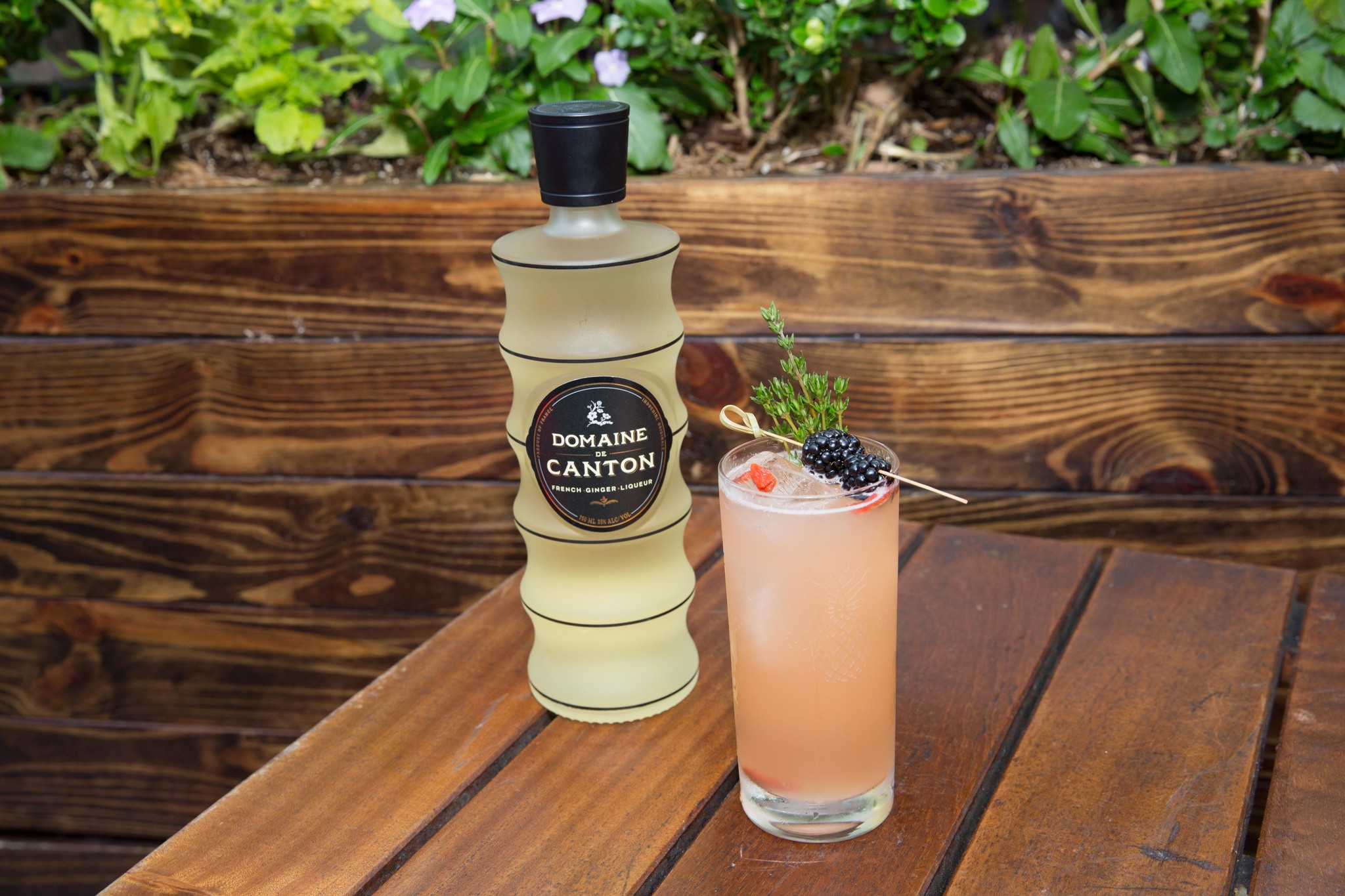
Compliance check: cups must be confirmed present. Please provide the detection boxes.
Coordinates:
[717,431,900,847]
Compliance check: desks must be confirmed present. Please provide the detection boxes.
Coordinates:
[97,490,1345,896]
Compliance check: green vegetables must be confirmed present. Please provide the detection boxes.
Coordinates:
[748,301,850,469]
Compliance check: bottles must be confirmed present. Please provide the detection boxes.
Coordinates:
[489,99,701,726]
